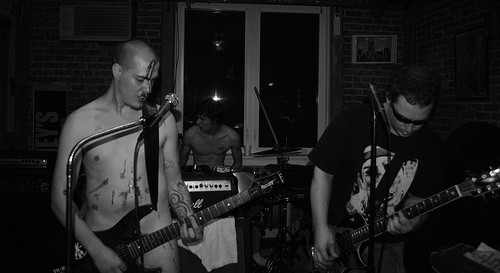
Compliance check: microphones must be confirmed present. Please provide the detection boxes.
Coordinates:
[369,82,390,128]
[138,93,179,141]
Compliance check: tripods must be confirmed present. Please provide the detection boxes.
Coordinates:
[264,159,298,273]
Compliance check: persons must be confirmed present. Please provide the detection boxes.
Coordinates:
[308,63,447,273]
[179,97,273,270]
[51,39,204,273]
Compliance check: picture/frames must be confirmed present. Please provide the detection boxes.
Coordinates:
[352,34,398,64]
[452,26,489,103]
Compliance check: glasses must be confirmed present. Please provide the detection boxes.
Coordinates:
[391,103,429,125]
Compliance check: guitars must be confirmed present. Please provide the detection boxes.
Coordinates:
[273,164,500,273]
[42,163,290,273]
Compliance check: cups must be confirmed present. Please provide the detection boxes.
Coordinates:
[243,145,251,156]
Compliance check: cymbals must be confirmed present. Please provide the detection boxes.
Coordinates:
[252,147,302,155]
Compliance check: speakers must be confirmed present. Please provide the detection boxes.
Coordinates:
[0,192,68,273]
